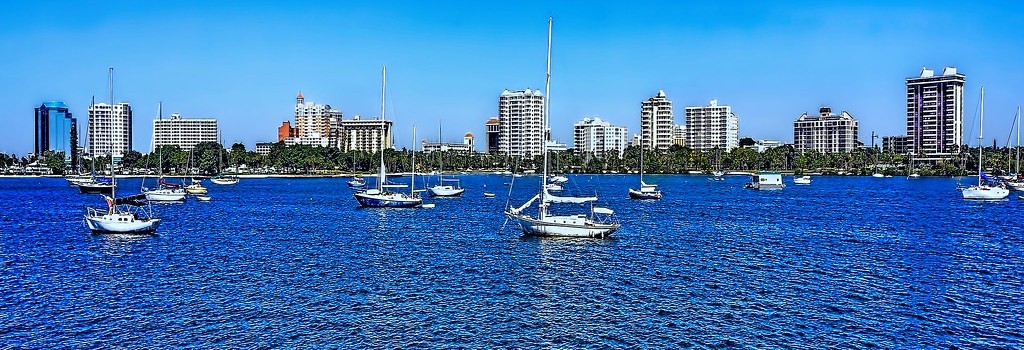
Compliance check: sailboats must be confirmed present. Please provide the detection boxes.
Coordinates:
[346,66,464,208]
[907,148,920,180]
[65,95,118,194]
[956,86,1024,200]
[629,148,662,199]
[494,18,620,240]
[140,101,211,201]
[83,67,162,235]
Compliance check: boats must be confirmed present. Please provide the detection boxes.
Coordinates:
[484,192,495,196]
[794,176,811,184]
[872,167,892,178]
[210,178,240,185]
